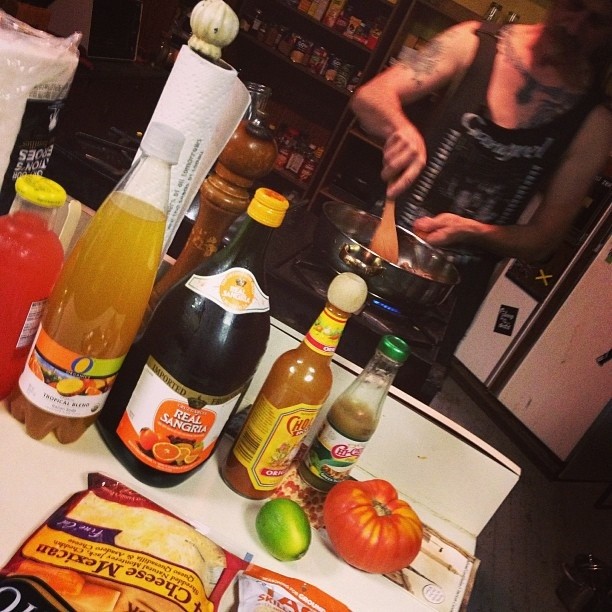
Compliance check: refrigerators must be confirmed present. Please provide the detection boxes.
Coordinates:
[439,166,612,481]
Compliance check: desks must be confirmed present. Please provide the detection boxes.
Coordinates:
[1,180,521,612]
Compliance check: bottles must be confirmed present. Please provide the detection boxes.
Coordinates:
[248,7,266,34]
[319,53,335,77]
[272,22,288,48]
[333,5,354,34]
[290,37,309,64]
[296,335,408,492]
[291,144,316,174]
[301,41,316,67]
[11,124,184,446]
[278,31,297,55]
[220,273,368,499]
[297,0,312,13]
[366,25,381,49]
[352,21,368,43]
[322,0,346,29]
[346,63,366,94]
[260,124,276,140]
[272,126,300,168]
[308,46,324,68]
[334,64,351,87]
[324,56,342,81]
[312,52,328,71]
[0,175,68,405]
[298,146,324,183]
[313,0,330,22]
[307,0,320,16]
[344,16,361,38]
[97,188,289,487]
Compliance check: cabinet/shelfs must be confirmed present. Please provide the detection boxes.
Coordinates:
[133,0,404,202]
[306,0,487,230]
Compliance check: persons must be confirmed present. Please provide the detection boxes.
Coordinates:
[350,2,612,335]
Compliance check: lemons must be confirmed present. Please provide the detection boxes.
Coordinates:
[256,498,311,562]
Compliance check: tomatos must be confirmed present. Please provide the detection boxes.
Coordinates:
[323,479,423,575]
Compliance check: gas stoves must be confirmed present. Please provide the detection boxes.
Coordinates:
[295,245,449,347]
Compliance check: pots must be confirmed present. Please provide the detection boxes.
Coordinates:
[321,202,498,319]
